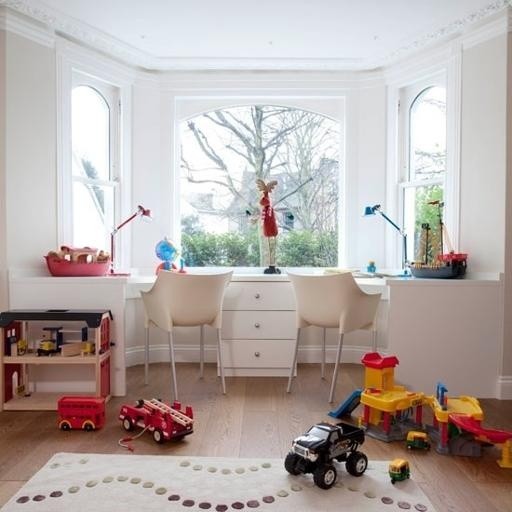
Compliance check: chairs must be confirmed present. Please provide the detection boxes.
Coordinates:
[139,270,234,404]
[285,271,383,404]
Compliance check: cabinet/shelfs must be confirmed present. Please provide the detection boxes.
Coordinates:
[0,309,113,412]
[218,274,298,377]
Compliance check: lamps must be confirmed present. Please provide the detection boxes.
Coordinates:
[108,204,153,276]
[364,204,412,277]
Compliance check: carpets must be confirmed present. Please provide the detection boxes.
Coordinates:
[0,452,439,512]
